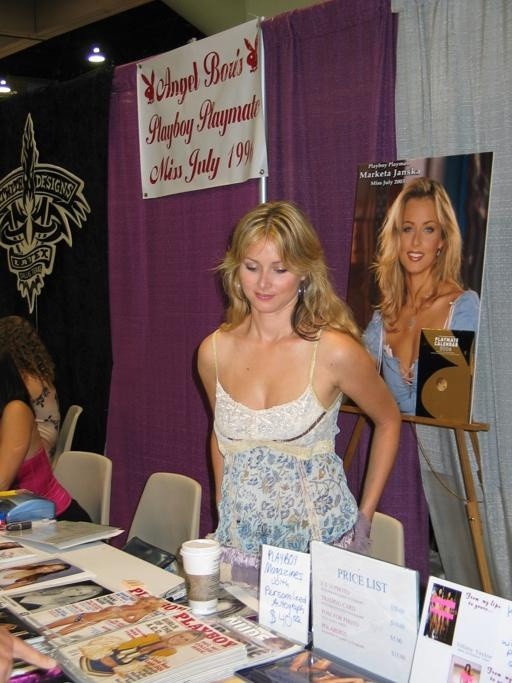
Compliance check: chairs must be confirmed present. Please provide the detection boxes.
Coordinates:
[123,472,203,561]
[52,450,113,544]
[51,404,83,471]
[366,511,406,567]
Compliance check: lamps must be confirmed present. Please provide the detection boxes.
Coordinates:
[87,45,107,62]
[0,79,11,93]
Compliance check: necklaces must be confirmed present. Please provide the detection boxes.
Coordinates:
[406,304,427,329]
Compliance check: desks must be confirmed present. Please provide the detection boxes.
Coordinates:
[0,531,185,602]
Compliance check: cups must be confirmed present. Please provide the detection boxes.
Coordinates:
[178,537,221,617]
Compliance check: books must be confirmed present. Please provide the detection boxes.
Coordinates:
[1,520,393,682]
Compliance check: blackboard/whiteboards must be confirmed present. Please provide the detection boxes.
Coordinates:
[257,540,511,682]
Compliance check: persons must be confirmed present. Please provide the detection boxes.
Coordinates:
[362,174,480,417]
[0,314,62,462]
[0,349,94,523]
[457,662,474,682]
[195,200,403,586]
[439,591,452,634]
[432,587,444,636]
